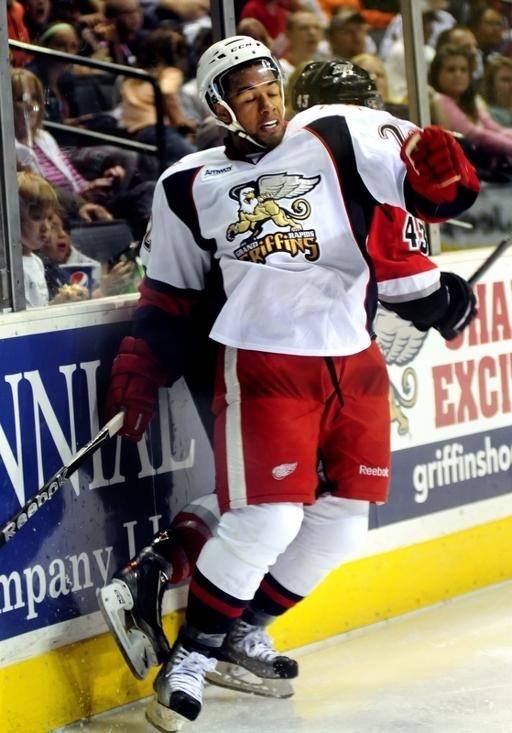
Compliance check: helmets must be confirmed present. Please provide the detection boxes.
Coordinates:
[292,57,384,112]
[196,35,287,102]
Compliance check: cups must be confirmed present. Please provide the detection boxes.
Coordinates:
[59,263,93,301]
[122,248,146,293]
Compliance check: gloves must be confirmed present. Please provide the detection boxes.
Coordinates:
[107,335,161,442]
[432,269,476,345]
[399,126,481,218]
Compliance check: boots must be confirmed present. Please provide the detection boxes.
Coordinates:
[111,526,175,664]
[152,627,219,722]
[222,607,299,679]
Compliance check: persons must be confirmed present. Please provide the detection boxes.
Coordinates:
[10,1,511,311]
